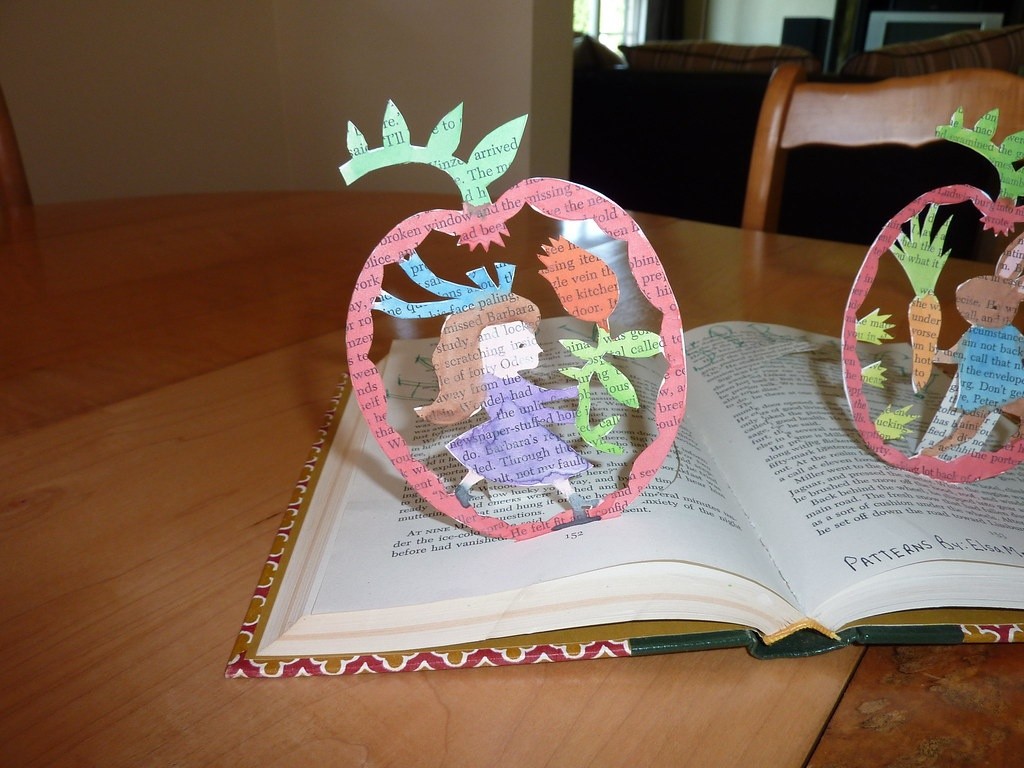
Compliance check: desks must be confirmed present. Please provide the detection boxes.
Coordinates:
[0,192,1022,768]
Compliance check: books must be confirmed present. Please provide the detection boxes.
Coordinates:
[220,306,1024,679]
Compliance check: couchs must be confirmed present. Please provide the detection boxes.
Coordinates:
[571,64,1023,260]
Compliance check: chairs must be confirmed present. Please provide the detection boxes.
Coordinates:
[742,60,1024,237]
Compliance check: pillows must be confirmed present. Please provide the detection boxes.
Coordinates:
[571,30,622,65]
[838,24,1023,81]
[619,38,823,80]
